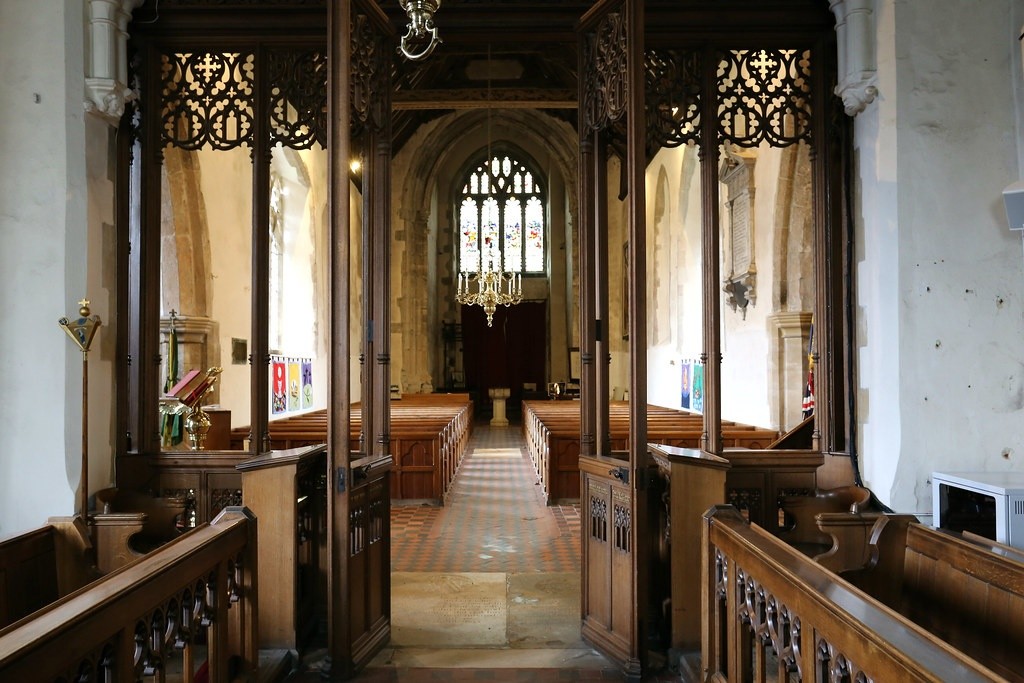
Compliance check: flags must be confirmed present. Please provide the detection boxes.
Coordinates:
[801,313,815,422]
[160,329,184,446]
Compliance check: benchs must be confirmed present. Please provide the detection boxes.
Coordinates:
[522,400,779,505]
[229,393,471,505]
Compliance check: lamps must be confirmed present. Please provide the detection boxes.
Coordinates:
[454,45,540,391]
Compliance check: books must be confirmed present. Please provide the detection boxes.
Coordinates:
[165,370,216,407]
